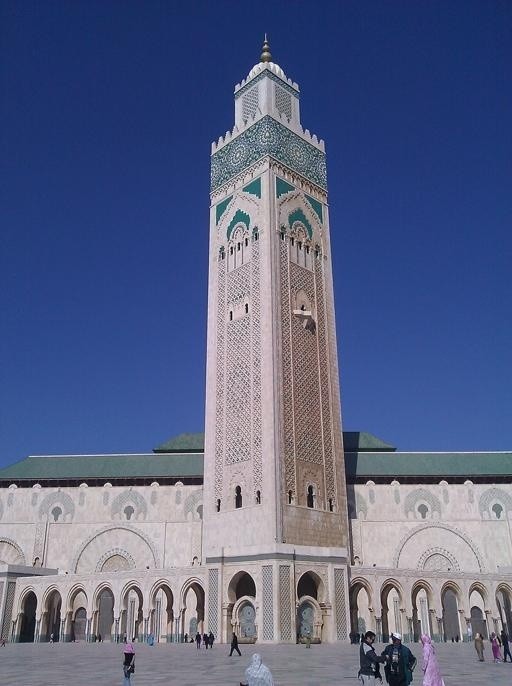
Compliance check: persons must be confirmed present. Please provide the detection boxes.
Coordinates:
[350,631,445,686]
[305,630,312,648]
[147,632,155,646]
[97,633,102,642]
[245,653,275,686]
[474,630,511,662]
[229,632,242,656]
[1,637,6,648]
[119,644,135,686]
[48,633,56,647]
[124,632,128,643]
[185,632,215,649]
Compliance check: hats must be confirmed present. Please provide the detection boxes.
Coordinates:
[390,632,402,640]
